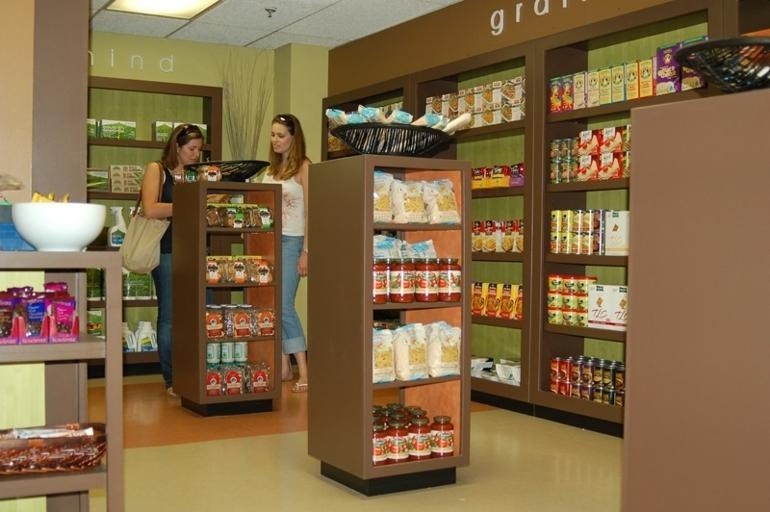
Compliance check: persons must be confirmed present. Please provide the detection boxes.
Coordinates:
[262,113,313,393]
[141,123,204,398]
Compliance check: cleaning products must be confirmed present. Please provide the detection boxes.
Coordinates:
[107,206,129,247]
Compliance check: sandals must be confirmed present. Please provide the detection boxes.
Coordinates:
[292,382,308,393]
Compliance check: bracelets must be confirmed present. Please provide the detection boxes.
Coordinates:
[300,248,309,254]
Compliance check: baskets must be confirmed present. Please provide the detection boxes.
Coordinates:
[330,122,450,157]
[674,37,770,94]
[184,160,270,181]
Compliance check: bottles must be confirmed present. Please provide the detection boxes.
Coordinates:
[206,303,275,340]
[206,362,274,397]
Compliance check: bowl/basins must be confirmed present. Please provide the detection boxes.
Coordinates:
[11,202,107,253]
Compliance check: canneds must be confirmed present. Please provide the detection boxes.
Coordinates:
[546,274,597,330]
[206,254,273,284]
[372,404,454,462]
[548,207,606,255]
[372,259,463,305]
[551,139,579,186]
[206,201,273,229]
[206,304,274,338]
[548,352,630,409]
[206,342,248,365]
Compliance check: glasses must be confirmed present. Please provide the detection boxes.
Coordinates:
[180,125,199,138]
[277,114,293,127]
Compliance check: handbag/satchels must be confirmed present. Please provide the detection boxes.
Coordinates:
[119,211,172,275]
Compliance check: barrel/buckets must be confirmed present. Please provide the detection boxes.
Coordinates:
[122,318,158,353]
